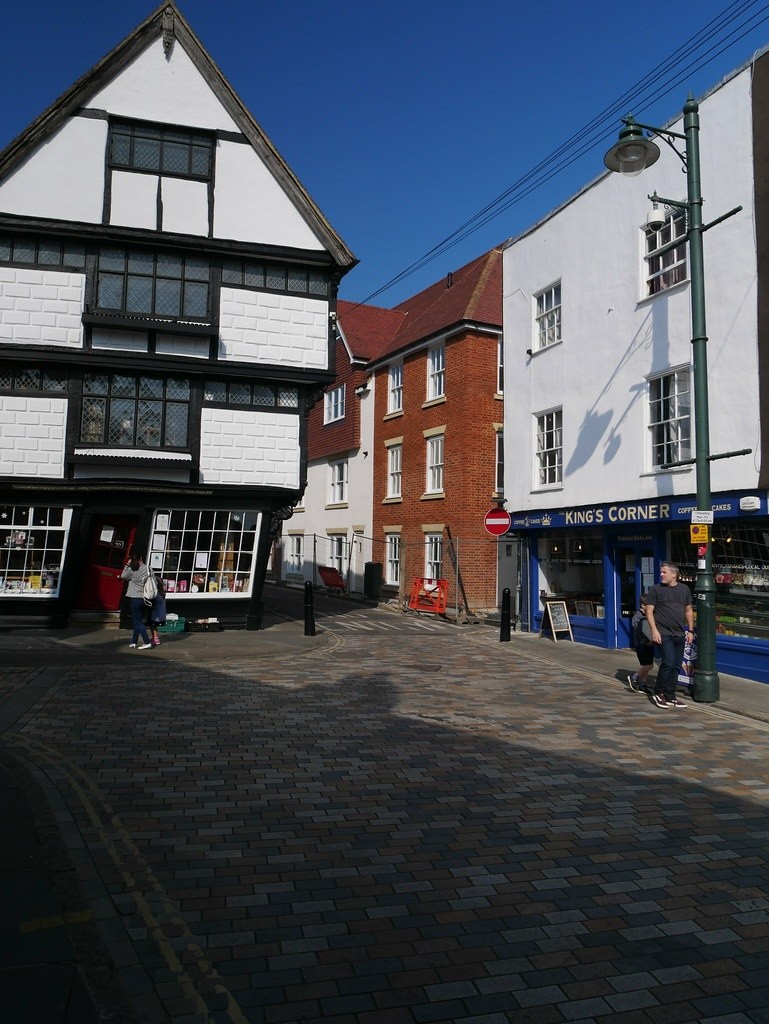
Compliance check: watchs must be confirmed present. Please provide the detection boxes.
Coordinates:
[688,630,695,635]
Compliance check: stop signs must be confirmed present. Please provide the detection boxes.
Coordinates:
[483,507,512,536]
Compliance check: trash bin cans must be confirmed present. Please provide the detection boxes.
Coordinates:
[364,562,382,598]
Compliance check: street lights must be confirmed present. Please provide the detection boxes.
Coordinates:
[601,87,726,704]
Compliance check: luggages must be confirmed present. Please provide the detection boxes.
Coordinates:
[152,637,160,644]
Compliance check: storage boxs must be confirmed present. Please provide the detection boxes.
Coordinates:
[218,560,234,580]
[219,542,233,560]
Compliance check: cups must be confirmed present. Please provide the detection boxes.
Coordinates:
[739,617,745,622]
[745,618,750,623]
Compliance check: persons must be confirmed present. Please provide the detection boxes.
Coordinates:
[121,550,154,650]
[645,561,694,709]
[145,576,166,645]
[626,592,656,695]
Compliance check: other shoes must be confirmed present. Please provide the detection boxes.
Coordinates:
[638,684,654,694]
[627,675,637,693]
[666,698,687,707]
[129,643,137,648]
[652,694,669,709]
[138,643,152,650]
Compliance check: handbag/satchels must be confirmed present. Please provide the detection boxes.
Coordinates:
[143,566,158,606]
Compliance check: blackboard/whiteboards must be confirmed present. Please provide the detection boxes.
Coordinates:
[541,601,573,631]
